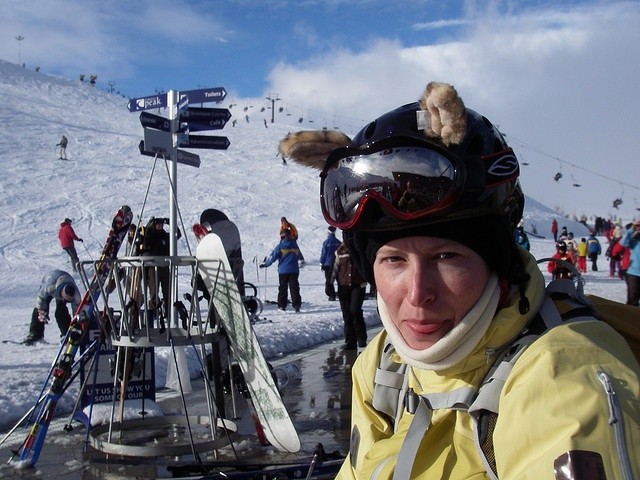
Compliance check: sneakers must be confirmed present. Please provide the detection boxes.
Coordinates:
[343,344,356,349]
[329,296,334,300]
[61,336,67,344]
[359,340,365,347]
[26,332,44,342]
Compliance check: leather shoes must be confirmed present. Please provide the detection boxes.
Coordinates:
[105,278,116,293]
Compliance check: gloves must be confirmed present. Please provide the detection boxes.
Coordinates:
[298,263,302,267]
[38,309,50,324]
[259,262,266,268]
[320,262,323,272]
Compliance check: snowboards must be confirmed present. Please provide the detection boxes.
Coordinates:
[200,209,230,419]
[211,220,257,436]
[193,232,302,453]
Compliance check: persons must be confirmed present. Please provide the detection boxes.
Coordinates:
[56,135,68,159]
[59,218,84,273]
[25,268,82,345]
[280,217,298,241]
[320,225,342,302]
[259,228,305,314]
[142,215,182,319]
[547,218,640,305]
[278,82,639,479]
[328,240,368,350]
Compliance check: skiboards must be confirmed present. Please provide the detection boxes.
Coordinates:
[17,204,133,468]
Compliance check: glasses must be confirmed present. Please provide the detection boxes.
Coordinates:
[559,243,564,246]
[319,134,520,229]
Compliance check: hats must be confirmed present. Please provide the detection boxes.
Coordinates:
[556,241,567,253]
[328,226,335,233]
[128,224,136,228]
[280,229,290,238]
[63,282,75,302]
[277,81,531,315]
